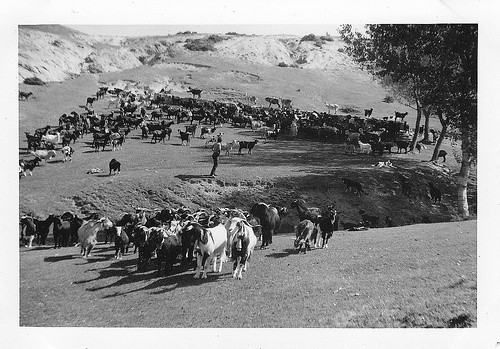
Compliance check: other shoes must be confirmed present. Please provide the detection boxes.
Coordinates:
[211,174,215,177]
[114,171,117,174]
[118,171,120,173]
[214,173,218,175]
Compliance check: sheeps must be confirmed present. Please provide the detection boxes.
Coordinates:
[428,181,442,204]
[400,179,411,196]
[342,178,364,197]
[19,198,399,281]
[19,85,448,178]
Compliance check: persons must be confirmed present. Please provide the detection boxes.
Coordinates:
[209,137,222,178]
